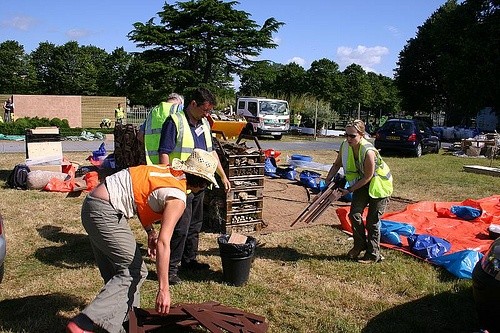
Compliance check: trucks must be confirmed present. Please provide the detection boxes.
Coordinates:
[211,96,294,140]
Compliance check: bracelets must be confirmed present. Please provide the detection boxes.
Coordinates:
[347,187,353,193]
[146,228,154,234]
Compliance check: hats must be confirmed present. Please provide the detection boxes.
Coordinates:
[171,148,219,189]
[168,93,182,104]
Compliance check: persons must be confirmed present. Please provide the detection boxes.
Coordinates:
[158,87,231,284]
[294,112,301,127]
[3,98,14,122]
[136,93,184,165]
[67,148,220,333]
[325,119,393,264]
[115,103,124,123]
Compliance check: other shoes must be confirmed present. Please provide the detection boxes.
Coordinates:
[347,244,365,259]
[169,273,181,285]
[358,256,375,264]
[66,315,96,333]
[182,258,210,271]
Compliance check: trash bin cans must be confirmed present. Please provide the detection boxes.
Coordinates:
[218,234,255,286]
[207,114,247,139]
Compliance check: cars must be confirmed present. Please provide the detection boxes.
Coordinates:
[373,118,442,158]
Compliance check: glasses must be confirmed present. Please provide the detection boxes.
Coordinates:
[345,133,360,138]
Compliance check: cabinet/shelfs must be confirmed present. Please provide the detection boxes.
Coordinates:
[208,146,268,233]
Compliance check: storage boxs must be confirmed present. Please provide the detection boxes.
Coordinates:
[28,164,62,172]
